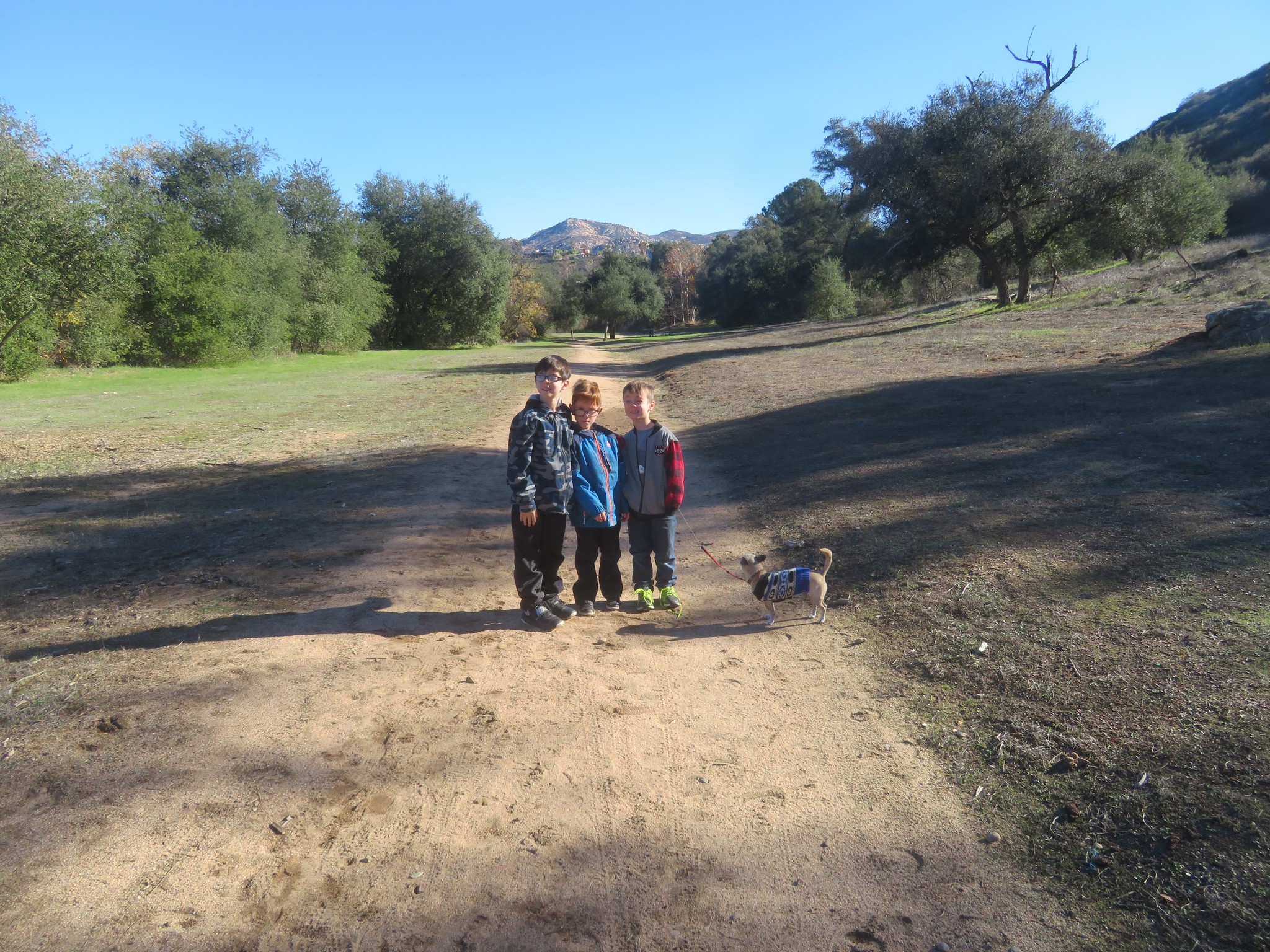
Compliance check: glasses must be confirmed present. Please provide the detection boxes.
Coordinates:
[535,375,565,382]
[572,404,600,417]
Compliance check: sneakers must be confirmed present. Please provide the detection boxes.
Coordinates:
[631,586,655,611]
[605,597,622,610]
[521,600,564,632]
[576,599,595,615]
[545,594,578,620]
[659,586,680,609]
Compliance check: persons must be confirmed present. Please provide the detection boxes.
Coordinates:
[507,356,576,631]
[568,378,626,616]
[622,381,685,611]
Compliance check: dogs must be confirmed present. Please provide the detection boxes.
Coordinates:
[740,548,833,626]
